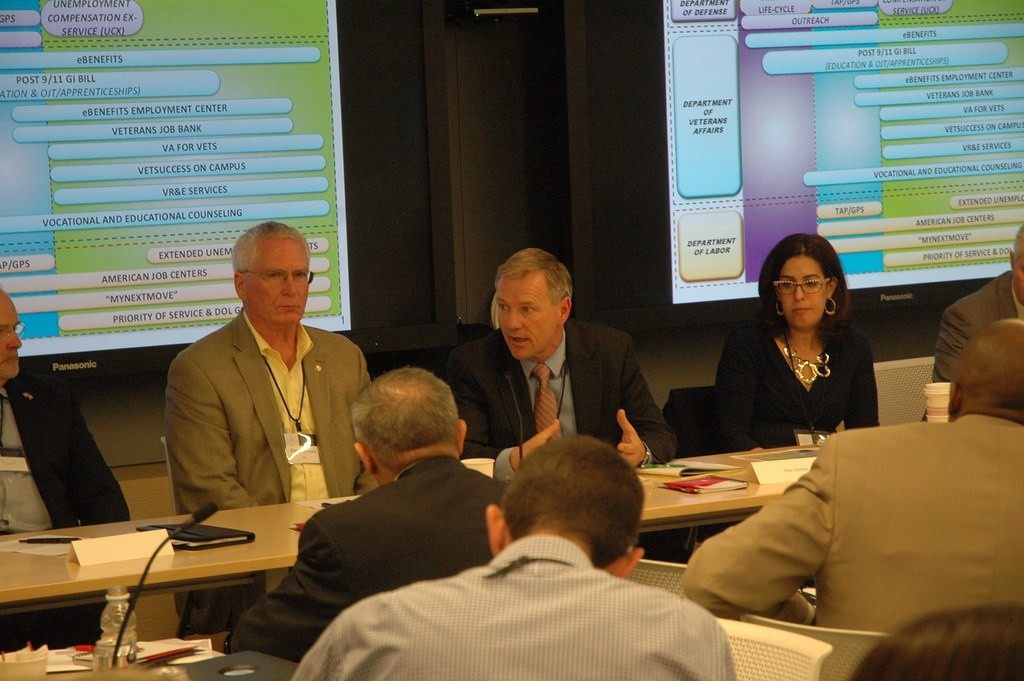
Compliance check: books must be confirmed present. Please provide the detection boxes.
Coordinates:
[139,523,256,551]
[666,476,748,494]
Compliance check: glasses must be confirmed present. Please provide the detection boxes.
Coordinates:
[773,279,828,294]
[0,324,24,337]
[241,269,313,287]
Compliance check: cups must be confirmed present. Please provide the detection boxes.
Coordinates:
[0,650,47,681]
[460,458,495,480]
[923,383,950,422]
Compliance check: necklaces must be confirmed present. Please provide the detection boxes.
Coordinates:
[784,345,831,384]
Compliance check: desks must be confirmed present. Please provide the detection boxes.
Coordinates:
[0,446,823,625]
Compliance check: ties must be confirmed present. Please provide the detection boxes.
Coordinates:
[532,364,557,433]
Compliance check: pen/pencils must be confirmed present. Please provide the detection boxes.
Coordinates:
[76,644,140,652]
[640,463,687,468]
[19,538,81,544]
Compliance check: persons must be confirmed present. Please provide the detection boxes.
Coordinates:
[227,369,508,663]
[294,434,738,681]
[446,247,680,467]
[713,233,879,453]
[685,319,1024,635]
[165,222,380,635]
[0,287,130,654]
[932,224,1024,384]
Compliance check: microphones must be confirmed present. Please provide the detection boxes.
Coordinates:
[112,501,220,666]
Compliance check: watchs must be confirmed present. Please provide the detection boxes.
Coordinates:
[641,452,649,468]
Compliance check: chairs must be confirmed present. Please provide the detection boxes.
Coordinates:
[876,349,943,425]
[623,555,890,680]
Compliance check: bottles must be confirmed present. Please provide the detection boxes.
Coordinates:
[101,584,137,642]
